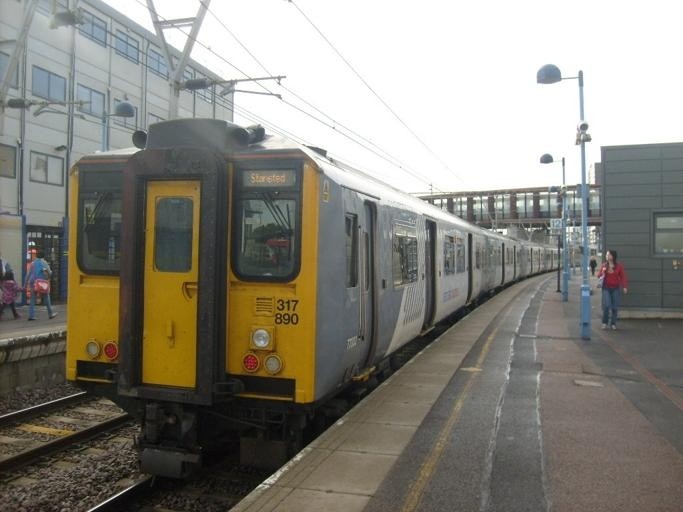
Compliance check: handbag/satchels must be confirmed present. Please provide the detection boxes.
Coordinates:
[33,277,51,295]
[596,274,605,288]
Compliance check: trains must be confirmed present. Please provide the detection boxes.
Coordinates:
[66,119,564,488]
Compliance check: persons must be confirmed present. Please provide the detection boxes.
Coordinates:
[589,255,597,277]
[597,248,629,330]
[0,268,26,319]
[23,250,58,321]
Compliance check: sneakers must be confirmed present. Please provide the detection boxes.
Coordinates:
[0,312,59,321]
[601,323,617,330]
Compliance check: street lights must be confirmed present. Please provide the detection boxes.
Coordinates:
[537,63,591,342]
[101,93,134,152]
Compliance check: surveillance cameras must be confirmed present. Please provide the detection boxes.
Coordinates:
[578,121,589,132]
